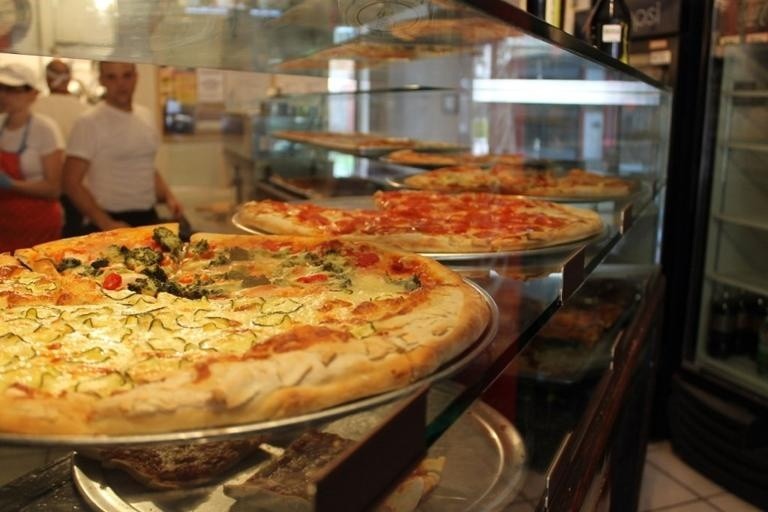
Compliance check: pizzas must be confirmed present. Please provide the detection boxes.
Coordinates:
[0,222,492,435]
[388,147,526,165]
[374,456,445,512]
[539,303,623,347]
[237,191,603,253]
[76,426,359,512]
[404,163,631,198]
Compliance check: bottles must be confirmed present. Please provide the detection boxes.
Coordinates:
[590,1,632,66]
[707,289,767,378]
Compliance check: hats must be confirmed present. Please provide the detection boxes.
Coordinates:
[0,64,42,93]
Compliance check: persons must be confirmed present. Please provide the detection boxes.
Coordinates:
[61,61,184,236]
[34,58,95,150]
[0,63,65,254]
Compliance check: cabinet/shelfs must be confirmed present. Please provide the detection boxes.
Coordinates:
[0,0,768,511]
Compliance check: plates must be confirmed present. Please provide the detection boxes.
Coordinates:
[0,275,502,447]
[231,193,612,261]
[71,380,531,511]
[386,170,650,203]
[504,278,643,386]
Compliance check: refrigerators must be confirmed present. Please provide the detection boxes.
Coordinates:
[667,0,768,512]
